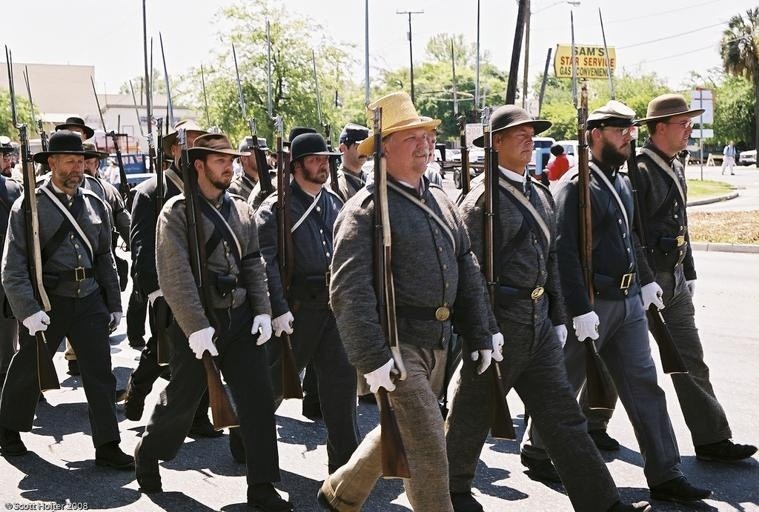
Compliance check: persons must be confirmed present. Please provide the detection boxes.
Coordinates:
[0,90,758,511]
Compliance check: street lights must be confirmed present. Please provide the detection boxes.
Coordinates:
[520,0,584,111]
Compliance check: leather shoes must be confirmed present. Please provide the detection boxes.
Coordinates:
[1,396,758,510]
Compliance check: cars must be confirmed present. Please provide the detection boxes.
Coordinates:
[686,141,758,167]
[9,123,589,196]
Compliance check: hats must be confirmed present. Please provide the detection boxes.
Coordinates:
[0,91,705,160]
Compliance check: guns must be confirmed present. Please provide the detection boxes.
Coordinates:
[1,1,690,479]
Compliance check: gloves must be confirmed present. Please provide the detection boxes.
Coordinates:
[21,279,696,393]
